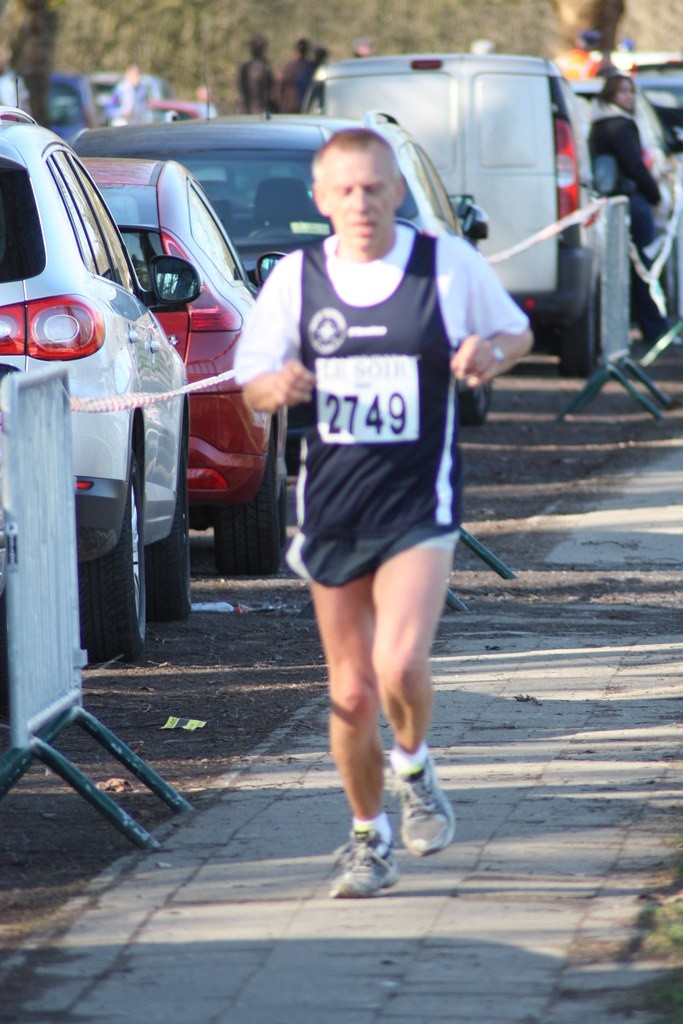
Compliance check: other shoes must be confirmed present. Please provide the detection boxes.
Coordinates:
[643,333,683,347]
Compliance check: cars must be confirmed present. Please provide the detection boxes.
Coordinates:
[70,159,296,586]
[45,75,210,131]
[552,48,683,153]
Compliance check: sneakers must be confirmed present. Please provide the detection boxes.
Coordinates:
[388,752,455,857]
[328,826,400,899]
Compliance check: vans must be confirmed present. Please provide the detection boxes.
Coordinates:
[298,52,611,381]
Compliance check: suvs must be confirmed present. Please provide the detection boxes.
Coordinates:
[56,106,499,424]
[1,107,201,657]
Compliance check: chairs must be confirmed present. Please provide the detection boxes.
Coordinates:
[252,176,314,227]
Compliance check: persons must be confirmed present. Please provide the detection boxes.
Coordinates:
[353,35,374,57]
[193,87,218,118]
[232,128,533,899]
[587,74,683,346]
[277,38,328,114]
[554,30,602,78]
[104,64,151,128]
[237,37,274,114]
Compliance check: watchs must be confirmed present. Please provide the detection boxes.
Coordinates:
[491,344,504,363]
